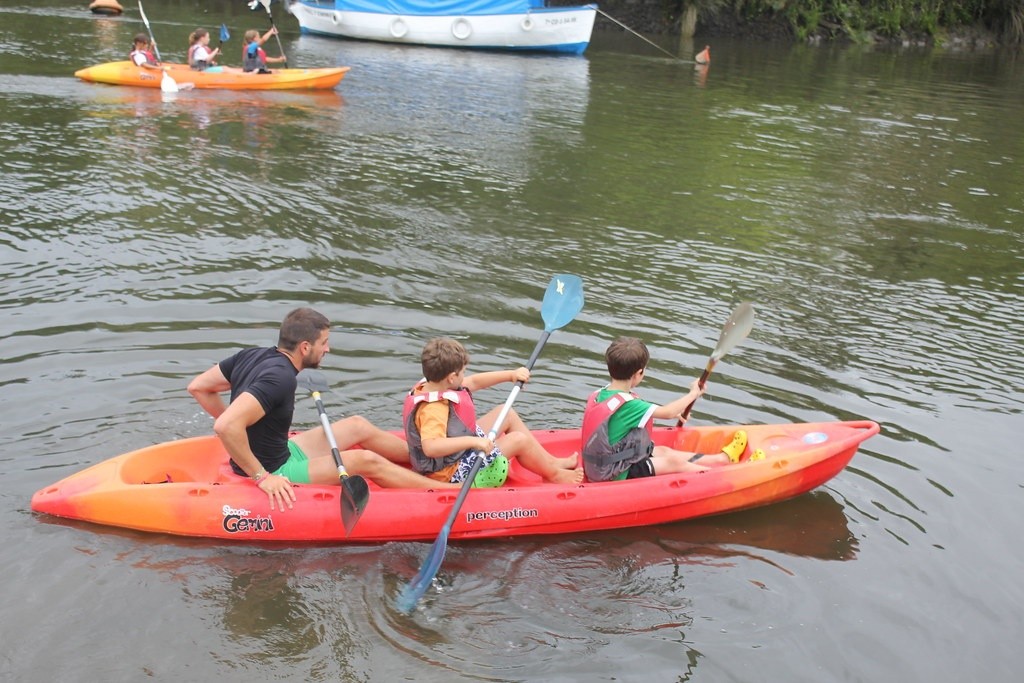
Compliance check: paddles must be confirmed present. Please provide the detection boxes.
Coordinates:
[677,301,755,427]
[137,0,178,93]
[304,381,369,538]
[261,0,289,68]
[396,273,584,613]
[214,21,232,64]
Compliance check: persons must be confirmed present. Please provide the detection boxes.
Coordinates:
[188,309,508,511]
[580,337,767,484]
[130,34,171,73]
[402,336,585,489]
[239,26,287,73]
[186,28,223,71]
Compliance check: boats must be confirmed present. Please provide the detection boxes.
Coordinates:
[24,419,882,541]
[290,0,599,56]
[70,57,352,89]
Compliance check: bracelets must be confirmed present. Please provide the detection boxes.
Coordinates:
[254,472,270,486]
[252,466,266,482]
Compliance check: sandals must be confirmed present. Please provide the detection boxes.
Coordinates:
[723,429,746,465]
[750,449,764,463]
[466,455,509,489]
